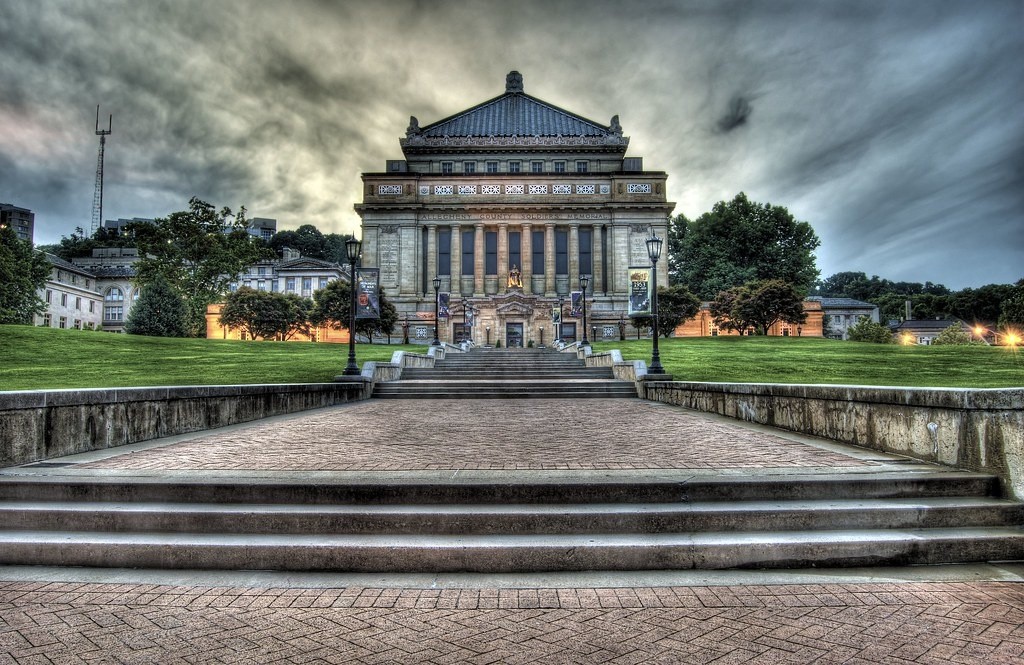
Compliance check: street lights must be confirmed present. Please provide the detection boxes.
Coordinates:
[462,296,468,342]
[431,273,442,345]
[341,230,363,375]
[644,227,667,374]
[579,273,590,344]
[558,296,565,342]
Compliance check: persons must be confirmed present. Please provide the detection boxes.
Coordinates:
[507,264,523,288]
